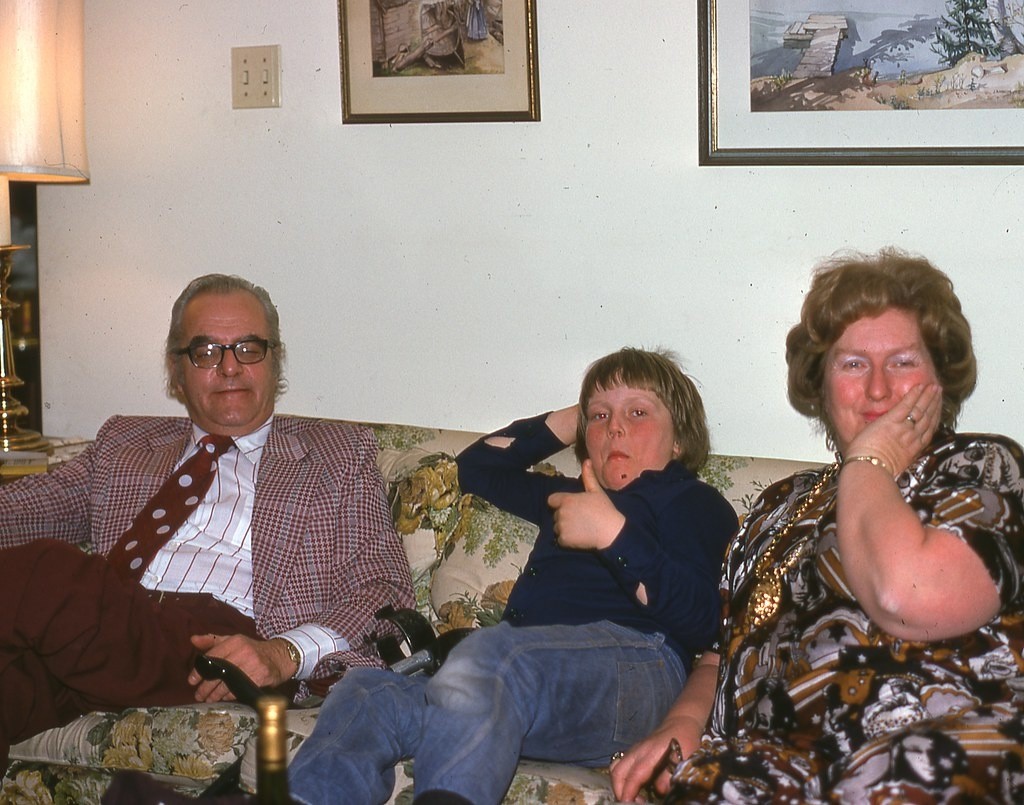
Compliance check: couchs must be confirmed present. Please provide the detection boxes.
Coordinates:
[0,423,833,805]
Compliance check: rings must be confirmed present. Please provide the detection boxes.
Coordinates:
[906,416,915,425]
[610,751,625,764]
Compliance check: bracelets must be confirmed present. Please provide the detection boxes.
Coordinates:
[842,455,887,468]
[285,640,301,675]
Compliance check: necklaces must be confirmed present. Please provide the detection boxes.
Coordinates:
[747,463,839,629]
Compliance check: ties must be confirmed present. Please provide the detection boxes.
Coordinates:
[105,435,232,584]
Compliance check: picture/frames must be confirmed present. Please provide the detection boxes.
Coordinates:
[698,0,1024,165]
[338,0,541,124]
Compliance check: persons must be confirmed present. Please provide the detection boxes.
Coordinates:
[609,250,1024,804]
[286,344,741,804]
[0,274,417,751]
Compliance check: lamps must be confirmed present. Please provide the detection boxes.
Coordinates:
[0,0,92,457]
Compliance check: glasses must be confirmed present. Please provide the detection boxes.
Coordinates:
[642,737,683,804]
[173,334,275,370]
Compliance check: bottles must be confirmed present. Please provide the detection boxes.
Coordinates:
[16,301,35,341]
